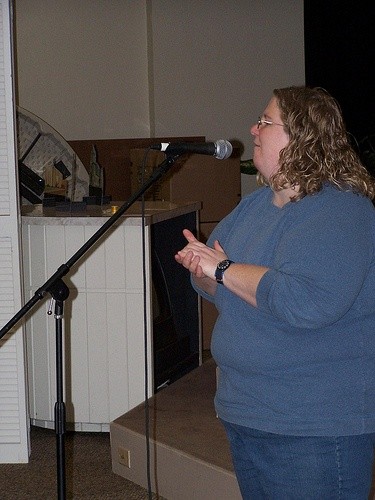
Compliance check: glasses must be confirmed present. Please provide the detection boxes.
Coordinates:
[256,117,290,132]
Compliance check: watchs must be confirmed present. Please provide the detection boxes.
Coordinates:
[215,260,235,285]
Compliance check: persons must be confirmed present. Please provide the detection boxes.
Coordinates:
[175,85,374,499]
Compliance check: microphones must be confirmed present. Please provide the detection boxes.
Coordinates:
[149,139,233,161]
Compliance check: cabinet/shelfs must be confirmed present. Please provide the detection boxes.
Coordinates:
[19,198,203,438]
